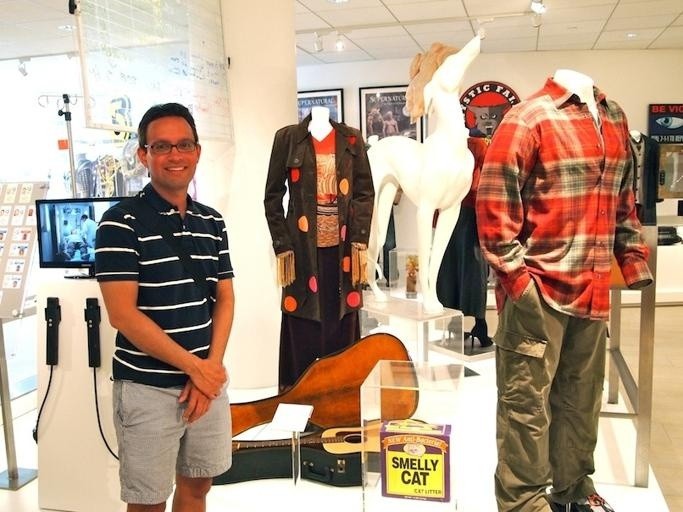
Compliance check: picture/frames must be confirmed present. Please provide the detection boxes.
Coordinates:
[297,88,344,123]
[358,85,423,144]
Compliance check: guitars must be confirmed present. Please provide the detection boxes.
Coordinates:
[231,418,429,456]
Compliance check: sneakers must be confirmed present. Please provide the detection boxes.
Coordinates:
[551,491,616,512]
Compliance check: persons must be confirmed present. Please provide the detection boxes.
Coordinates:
[436,124,491,350]
[628,127,660,225]
[264,104,375,398]
[61,228,91,260]
[382,111,401,139]
[93,102,235,512]
[80,215,97,248]
[61,218,70,233]
[478,67,656,512]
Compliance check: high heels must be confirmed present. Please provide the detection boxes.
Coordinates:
[448,315,494,349]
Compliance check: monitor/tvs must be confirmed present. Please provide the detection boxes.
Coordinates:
[36,197,135,278]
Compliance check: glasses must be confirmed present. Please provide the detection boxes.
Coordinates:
[145,138,197,153]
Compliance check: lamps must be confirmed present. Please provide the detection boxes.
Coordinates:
[294,0,547,53]
